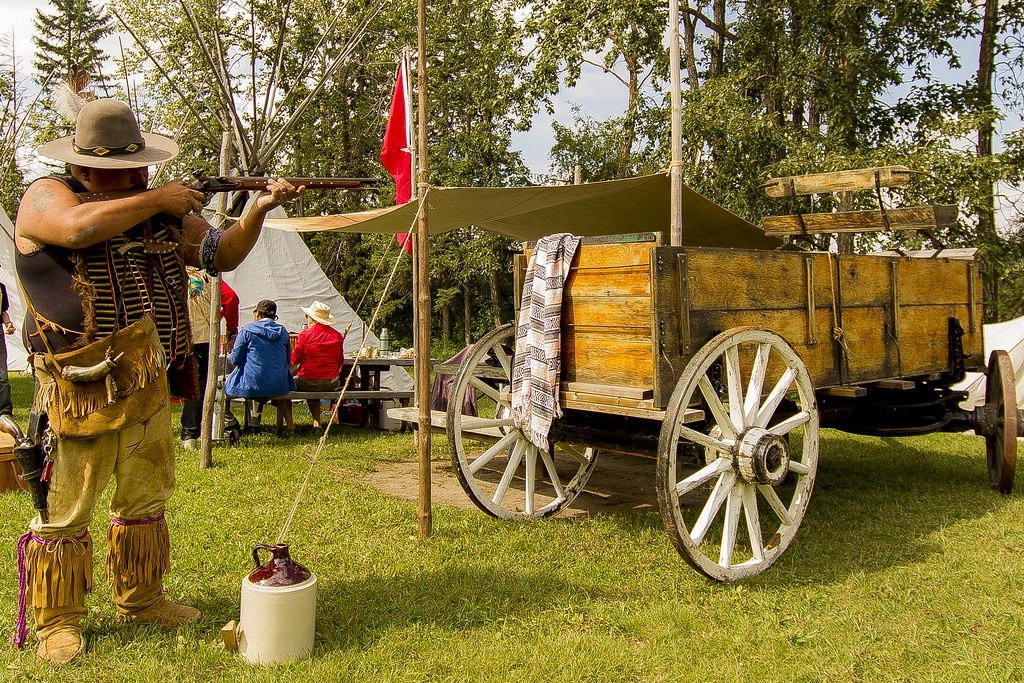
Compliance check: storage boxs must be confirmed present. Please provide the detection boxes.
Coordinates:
[0,430,28,493]
[340,402,364,426]
[379,401,403,431]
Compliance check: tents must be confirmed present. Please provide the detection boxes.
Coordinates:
[0,204,37,374]
[202,159,415,396]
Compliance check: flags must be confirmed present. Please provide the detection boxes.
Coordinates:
[376,56,417,254]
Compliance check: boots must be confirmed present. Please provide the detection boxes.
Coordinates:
[107,520,201,625]
[18,530,95,667]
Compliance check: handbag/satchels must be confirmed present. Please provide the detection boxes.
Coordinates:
[28,314,167,440]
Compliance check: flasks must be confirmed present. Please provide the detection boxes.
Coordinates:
[301,324,307,331]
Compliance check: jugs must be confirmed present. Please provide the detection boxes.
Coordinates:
[234,539,318,667]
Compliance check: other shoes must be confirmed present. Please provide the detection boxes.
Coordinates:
[279,426,294,436]
[312,424,324,435]
[183,436,197,451]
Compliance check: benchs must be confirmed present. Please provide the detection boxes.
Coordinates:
[243,390,414,435]
[386,405,510,444]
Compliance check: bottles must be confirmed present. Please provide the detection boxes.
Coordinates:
[380,326,389,358]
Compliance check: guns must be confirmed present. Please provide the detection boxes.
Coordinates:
[0,414,50,524]
[74,166,382,208]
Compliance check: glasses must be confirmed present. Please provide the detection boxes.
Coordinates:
[305,312,308,318]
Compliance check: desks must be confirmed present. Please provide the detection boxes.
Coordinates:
[343,358,438,427]
[431,364,509,382]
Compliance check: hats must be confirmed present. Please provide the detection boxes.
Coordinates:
[36,98,180,170]
[299,299,337,325]
[253,299,277,314]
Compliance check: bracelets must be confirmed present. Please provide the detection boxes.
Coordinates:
[6,322,12,325]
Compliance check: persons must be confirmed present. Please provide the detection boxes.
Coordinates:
[13,98,306,665]
[290,299,345,434]
[182,262,240,451]
[222,299,297,439]
[0,282,16,420]
